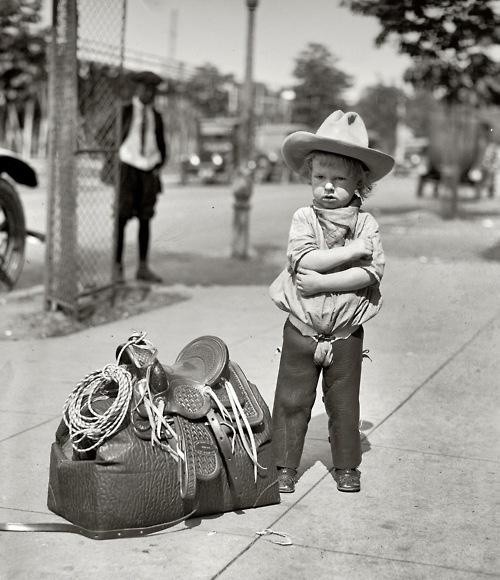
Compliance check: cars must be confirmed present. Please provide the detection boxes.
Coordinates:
[415,111,495,201]
[0,145,38,291]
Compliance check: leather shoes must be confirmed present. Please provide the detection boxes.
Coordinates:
[278,464,296,495]
[335,465,363,492]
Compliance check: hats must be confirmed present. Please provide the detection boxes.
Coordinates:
[279,109,396,184]
[129,71,164,87]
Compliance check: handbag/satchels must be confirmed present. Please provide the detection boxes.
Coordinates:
[0,326,283,541]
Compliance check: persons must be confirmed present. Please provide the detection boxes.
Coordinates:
[263,133,385,492]
[95,71,166,283]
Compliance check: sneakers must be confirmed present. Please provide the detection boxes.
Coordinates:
[112,259,165,285]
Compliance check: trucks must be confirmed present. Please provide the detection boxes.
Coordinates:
[177,113,318,186]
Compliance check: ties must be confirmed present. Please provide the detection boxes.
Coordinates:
[138,105,148,156]
